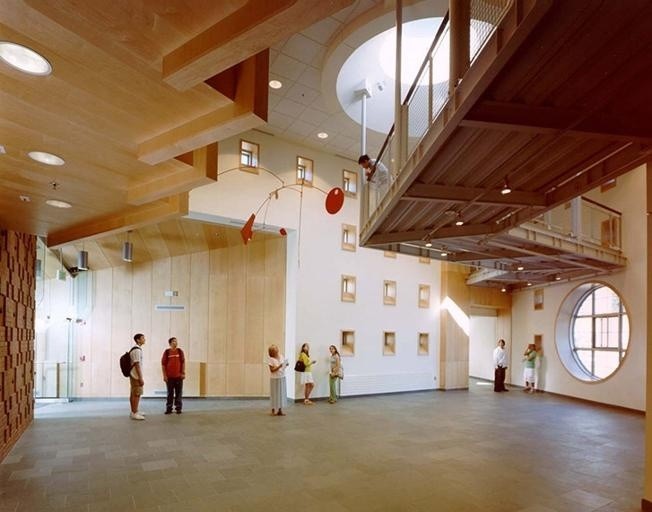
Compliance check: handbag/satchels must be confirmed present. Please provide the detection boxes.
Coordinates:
[333,367,343,379]
[295,361,305,372]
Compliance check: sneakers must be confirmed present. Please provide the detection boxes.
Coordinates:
[130,411,181,421]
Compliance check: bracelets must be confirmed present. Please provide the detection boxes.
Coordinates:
[182,372,185,375]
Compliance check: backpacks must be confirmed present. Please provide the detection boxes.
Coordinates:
[120,347,141,377]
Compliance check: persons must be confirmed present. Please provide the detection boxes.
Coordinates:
[328,345,340,404]
[130,334,146,419]
[162,338,185,414]
[299,343,316,405]
[269,346,290,416]
[359,154,393,197]
[523,344,536,392]
[494,339,509,392]
